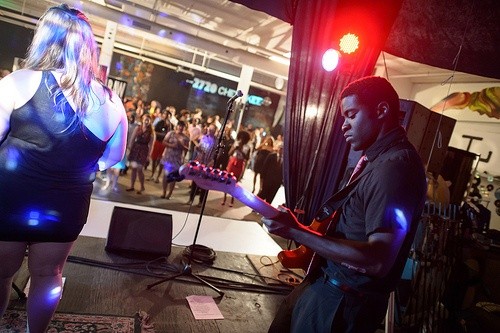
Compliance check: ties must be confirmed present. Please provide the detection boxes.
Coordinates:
[306,154,369,274]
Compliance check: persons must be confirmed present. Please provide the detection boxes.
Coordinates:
[0,3,129,333]
[261,75,426,333]
[118,97,284,216]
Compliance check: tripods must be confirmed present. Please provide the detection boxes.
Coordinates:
[144,105,238,297]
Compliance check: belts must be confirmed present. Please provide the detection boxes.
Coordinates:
[322,272,388,297]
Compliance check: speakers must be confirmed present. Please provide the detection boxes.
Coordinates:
[103,206,174,256]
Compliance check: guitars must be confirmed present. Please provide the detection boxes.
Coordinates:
[179,159,339,272]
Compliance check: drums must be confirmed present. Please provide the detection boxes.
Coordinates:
[413,212,455,260]
[396,258,446,316]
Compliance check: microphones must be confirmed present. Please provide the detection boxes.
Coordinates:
[226,90,243,104]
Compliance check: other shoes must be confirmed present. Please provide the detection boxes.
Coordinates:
[161,195,165,198]
[125,187,134,191]
[197,202,203,207]
[155,178,160,183]
[187,200,192,204]
[166,195,170,200]
[137,188,145,194]
[221,201,226,205]
[229,203,233,206]
[146,176,154,180]
